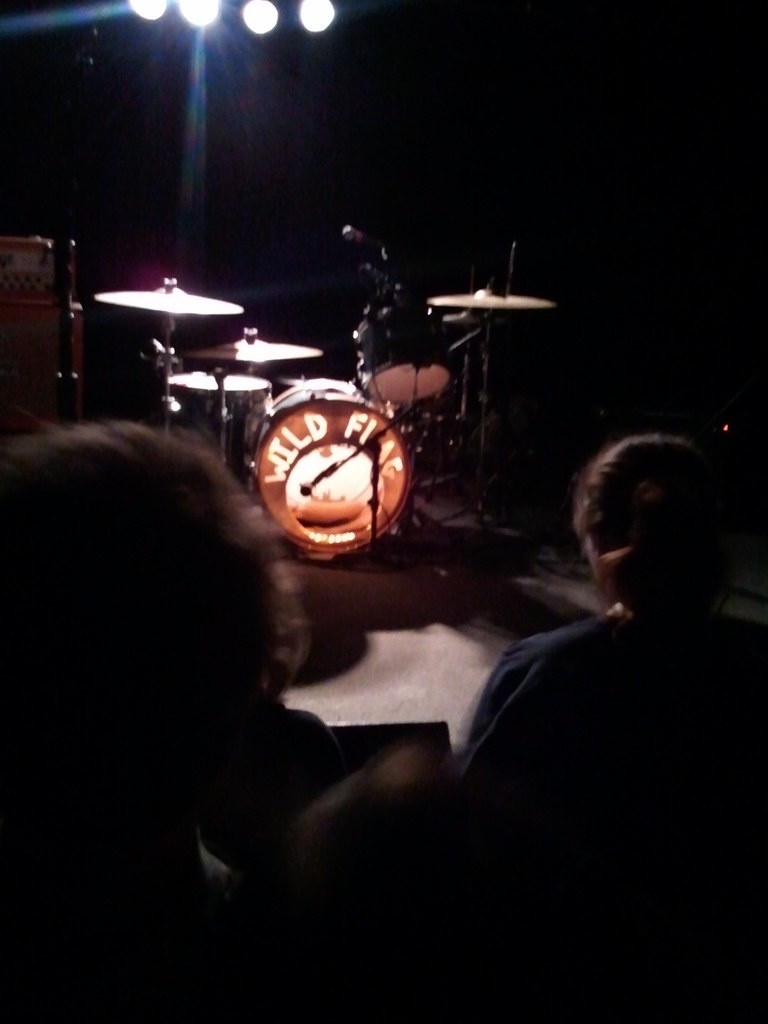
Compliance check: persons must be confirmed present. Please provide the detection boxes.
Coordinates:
[0,421,344,1024]
[458,433,768,802]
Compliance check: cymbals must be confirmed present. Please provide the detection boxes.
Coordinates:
[425,288,560,312]
[180,338,325,366]
[442,310,507,326]
[94,287,246,318]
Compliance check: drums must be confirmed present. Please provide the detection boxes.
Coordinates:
[350,302,452,405]
[167,370,271,471]
[249,379,415,557]
